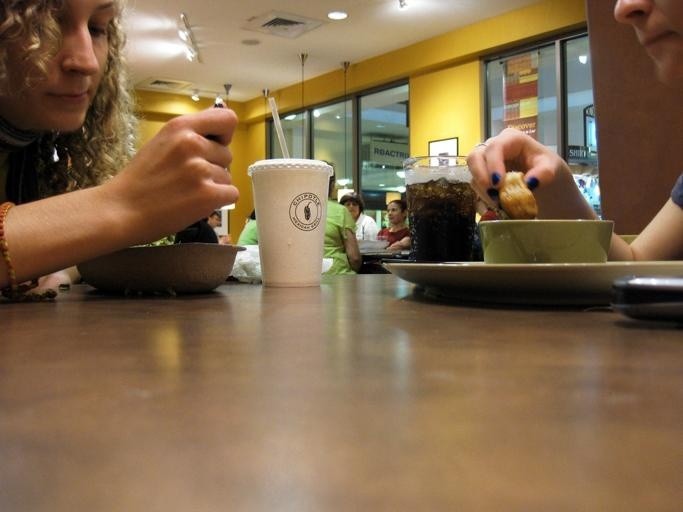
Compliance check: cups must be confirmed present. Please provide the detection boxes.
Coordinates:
[246,157,334,288]
[401,156,478,262]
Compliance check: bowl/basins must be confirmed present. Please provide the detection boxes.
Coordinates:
[74,246,244,293]
[477,218,615,263]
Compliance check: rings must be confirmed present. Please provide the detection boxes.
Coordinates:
[473,143,486,147]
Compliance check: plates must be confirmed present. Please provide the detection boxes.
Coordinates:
[381,261,562,307]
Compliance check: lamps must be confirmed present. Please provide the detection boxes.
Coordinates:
[178,13,203,63]
[337,61,355,202]
[220,84,235,210]
[188,89,230,104]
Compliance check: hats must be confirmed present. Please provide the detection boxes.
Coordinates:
[341,192,365,209]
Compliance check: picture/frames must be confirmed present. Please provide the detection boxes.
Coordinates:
[213,209,230,237]
[428,137,458,167]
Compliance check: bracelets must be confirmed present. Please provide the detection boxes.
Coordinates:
[0,201,36,292]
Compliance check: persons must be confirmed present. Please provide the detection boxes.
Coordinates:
[323,173,410,275]
[461,0,683,261]
[172,212,258,246]
[470,179,499,221]
[0,0,237,296]
